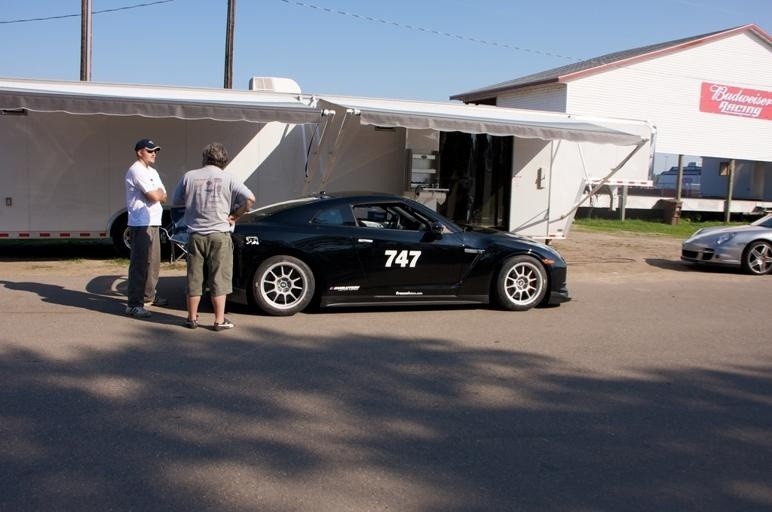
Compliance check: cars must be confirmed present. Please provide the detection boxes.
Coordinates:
[231,191,571,316]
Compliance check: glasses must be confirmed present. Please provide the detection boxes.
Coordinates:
[147,149,156,153]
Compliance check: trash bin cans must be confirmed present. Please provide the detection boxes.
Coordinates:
[665,200,683,225]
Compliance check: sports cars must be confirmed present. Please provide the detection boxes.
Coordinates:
[681,212,772,274]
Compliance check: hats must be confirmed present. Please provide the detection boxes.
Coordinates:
[134,138,162,153]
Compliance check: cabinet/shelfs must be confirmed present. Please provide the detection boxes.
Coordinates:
[408,146,443,193]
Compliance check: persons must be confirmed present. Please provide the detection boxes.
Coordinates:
[123,138,170,319]
[172,142,256,330]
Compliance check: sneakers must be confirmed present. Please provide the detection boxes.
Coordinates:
[186,317,199,329]
[213,321,235,332]
[126,306,152,318]
[143,296,168,307]
